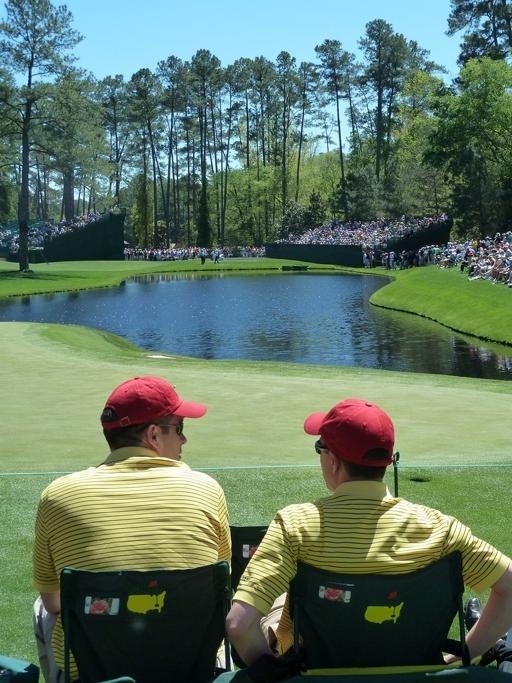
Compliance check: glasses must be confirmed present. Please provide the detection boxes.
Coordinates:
[314,440,328,454]
[157,422,183,435]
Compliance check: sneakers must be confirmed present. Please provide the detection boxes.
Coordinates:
[465,597,497,667]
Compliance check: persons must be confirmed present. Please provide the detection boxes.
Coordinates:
[32,375,233,683]
[0,204,118,254]
[224,398,512,668]
[464,596,512,676]
[124,243,266,266]
[274,211,512,288]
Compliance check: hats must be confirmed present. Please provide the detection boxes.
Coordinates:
[101,377,208,429]
[303,399,396,467]
